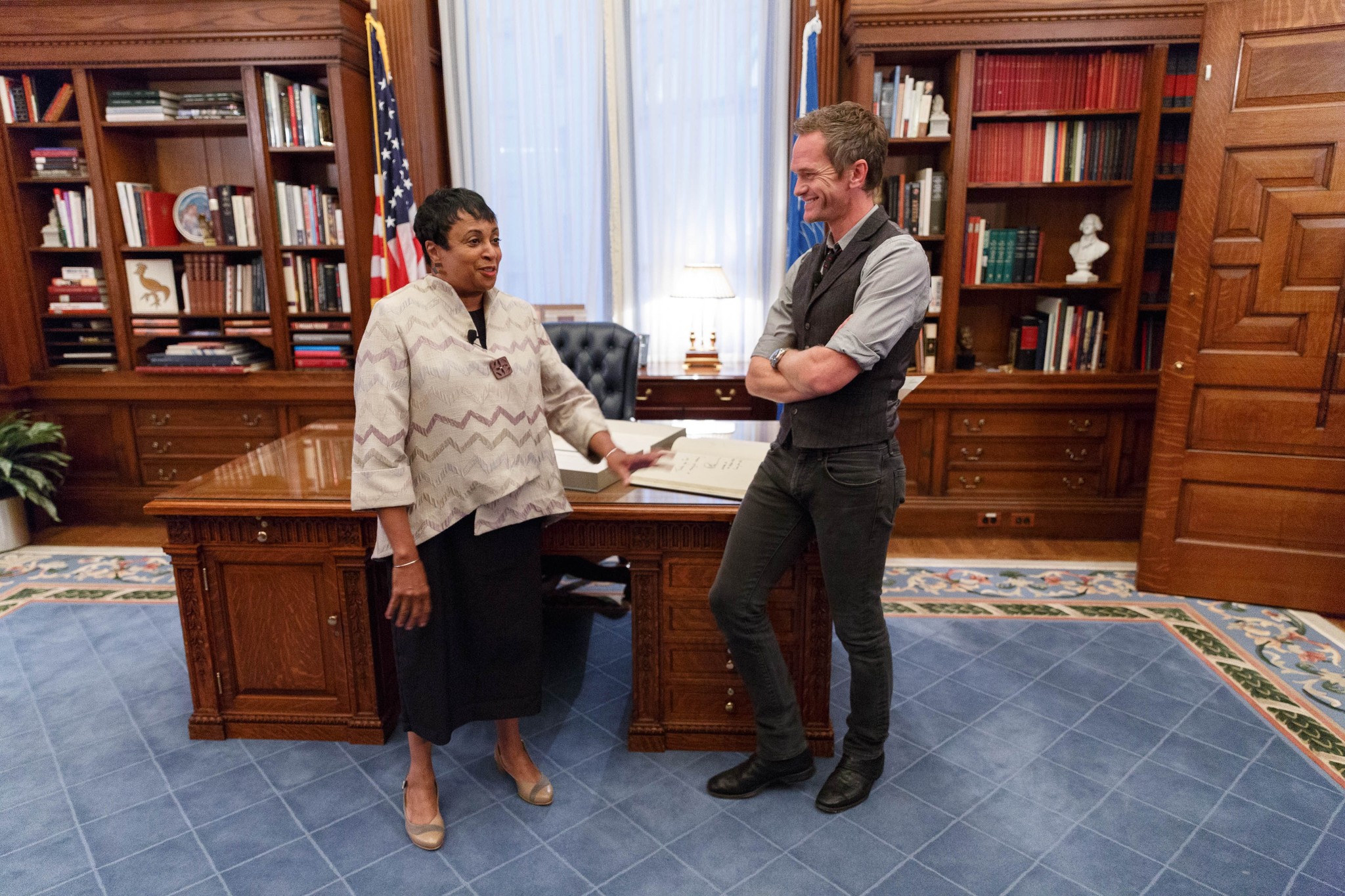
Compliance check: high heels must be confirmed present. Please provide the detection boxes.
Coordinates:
[403,774,444,849]
[493,739,554,805]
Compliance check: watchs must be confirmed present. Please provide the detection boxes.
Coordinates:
[769,347,790,367]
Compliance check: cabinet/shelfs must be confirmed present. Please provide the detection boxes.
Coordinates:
[850,33,1203,537]
[0,58,375,522]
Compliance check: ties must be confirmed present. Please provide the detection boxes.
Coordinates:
[823,246,842,276]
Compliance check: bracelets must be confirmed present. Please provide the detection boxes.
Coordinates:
[606,447,617,458]
[392,556,420,567]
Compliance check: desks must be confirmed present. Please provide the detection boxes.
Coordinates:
[143,410,837,757]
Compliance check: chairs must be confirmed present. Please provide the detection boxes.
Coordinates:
[539,320,640,421]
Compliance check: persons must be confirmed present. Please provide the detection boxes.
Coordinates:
[1068,213,1110,264]
[705,103,932,813]
[350,187,675,852]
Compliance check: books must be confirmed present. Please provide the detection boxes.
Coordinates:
[906,252,944,375]
[261,72,346,245]
[883,166,947,236]
[968,42,1201,185]
[0,74,74,124]
[963,214,1047,283]
[1012,295,1111,373]
[872,64,934,139]
[129,319,274,374]
[184,252,352,315]
[105,89,246,120]
[114,169,260,247]
[47,266,119,372]
[290,320,353,371]
[1138,43,1199,370]
[30,146,97,248]
[549,416,772,500]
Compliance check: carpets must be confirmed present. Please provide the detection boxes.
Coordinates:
[0,549,1345,895]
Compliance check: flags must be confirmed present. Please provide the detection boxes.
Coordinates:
[776,14,828,425]
[366,14,427,310]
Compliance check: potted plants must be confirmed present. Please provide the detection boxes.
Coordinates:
[0,414,74,552]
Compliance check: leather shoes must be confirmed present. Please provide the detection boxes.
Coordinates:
[707,752,815,799]
[815,753,885,814]
